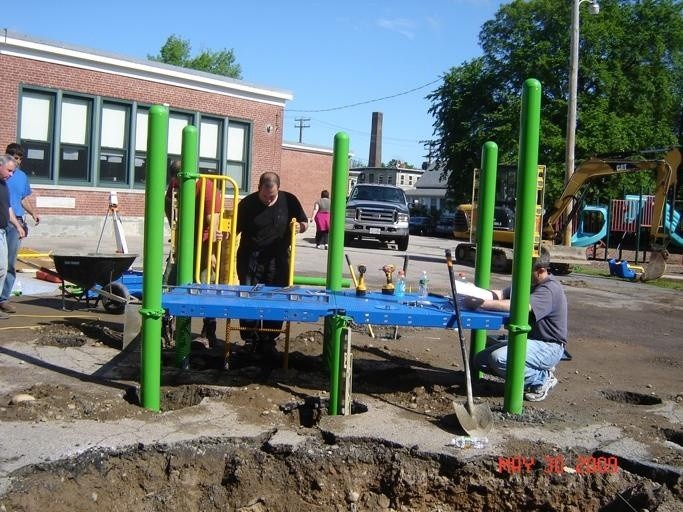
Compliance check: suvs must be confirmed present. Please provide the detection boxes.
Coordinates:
[344,183,412,252]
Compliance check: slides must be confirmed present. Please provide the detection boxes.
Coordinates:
[571,221,608,247]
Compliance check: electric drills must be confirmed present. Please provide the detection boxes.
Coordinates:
[356,264,366,296]
[378,264,395,295]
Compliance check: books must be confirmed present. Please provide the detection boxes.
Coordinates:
[453,279,495,305]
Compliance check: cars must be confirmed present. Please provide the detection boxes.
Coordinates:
[431,216,454,238]
[408,216,433,238]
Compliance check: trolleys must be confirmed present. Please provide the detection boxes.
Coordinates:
[16,250,136,313]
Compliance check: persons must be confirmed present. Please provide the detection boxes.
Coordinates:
[309,189,330,251]
[0,153,26,320]
[0,141,41,315]
[161,157,224,353]
[462,244,568,402]
[213,171,307,361]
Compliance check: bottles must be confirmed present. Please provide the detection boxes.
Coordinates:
[419,271,430,300]
[456,272,467,309]
[394,270,406,298]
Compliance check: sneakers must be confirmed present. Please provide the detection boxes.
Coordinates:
[240,338,279,376]
[523,366,558,402]
[0,301,16,318]
[316,244,329,249]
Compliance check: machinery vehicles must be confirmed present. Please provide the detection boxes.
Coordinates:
[451,145,682,282]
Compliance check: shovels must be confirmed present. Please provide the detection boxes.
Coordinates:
[445,249,494,437]
